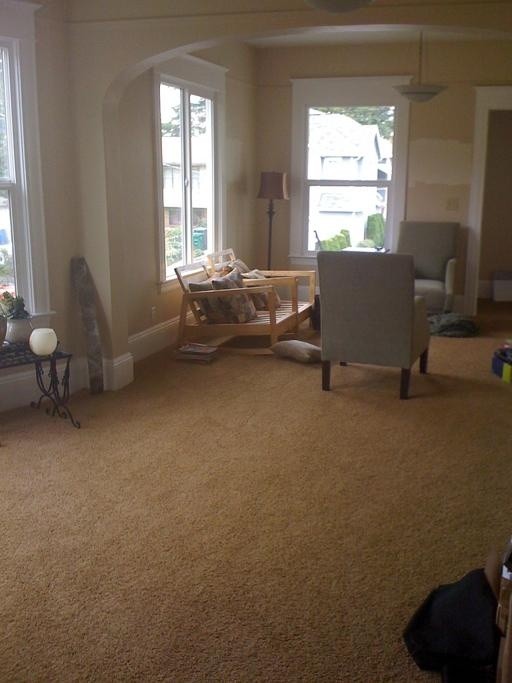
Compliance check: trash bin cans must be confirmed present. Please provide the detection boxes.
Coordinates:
[491,270,512,302]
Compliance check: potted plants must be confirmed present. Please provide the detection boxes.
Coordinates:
[0,292,34,346]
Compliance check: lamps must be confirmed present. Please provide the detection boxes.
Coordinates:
[392,30,448,105]
[256,170,290,277]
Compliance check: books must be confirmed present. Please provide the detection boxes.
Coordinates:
[176,343,219,361]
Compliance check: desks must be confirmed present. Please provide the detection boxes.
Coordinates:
[0,349,81,432]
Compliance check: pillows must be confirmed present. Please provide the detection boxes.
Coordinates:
[189,259,279,323]
[270,340,321,364]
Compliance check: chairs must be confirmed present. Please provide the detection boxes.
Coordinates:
[317,220,460,401]
[174,248,316,349]
